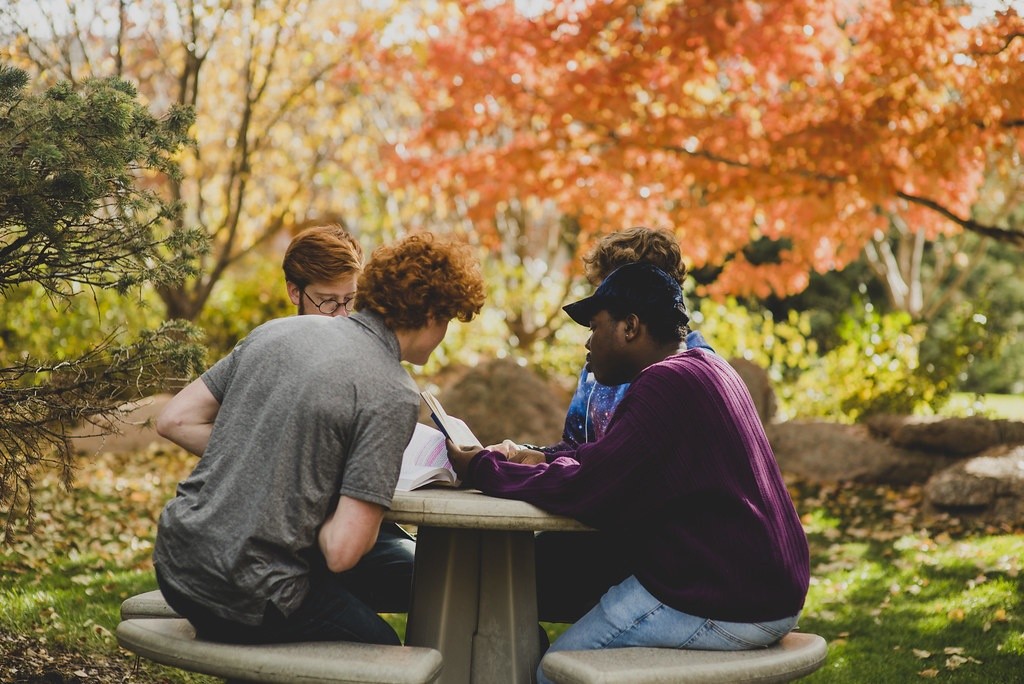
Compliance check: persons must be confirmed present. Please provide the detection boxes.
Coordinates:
[279,224,426,614]
[443,261,813,684]
[485,224,717,663]
[145,231,485,646]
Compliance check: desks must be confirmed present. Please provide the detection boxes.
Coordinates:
[381,489,601,684]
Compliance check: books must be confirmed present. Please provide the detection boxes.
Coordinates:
[389,420,468,492]
[420,390,486,454]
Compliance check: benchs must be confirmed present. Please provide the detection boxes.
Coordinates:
[541,631,829,684]
[116,619,444,684]
[120,591,185,622]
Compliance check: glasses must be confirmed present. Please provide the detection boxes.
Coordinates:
[303,291,357,315]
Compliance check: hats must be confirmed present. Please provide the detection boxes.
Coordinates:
[562,261,688,328]
